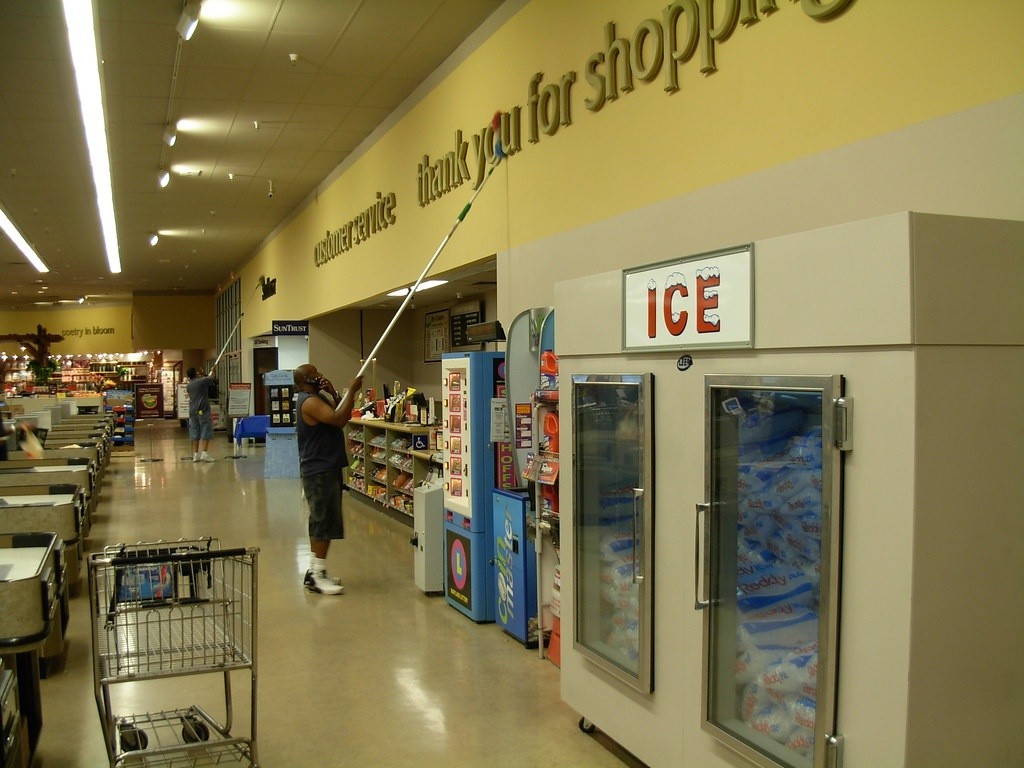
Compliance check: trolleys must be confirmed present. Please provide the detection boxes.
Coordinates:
[86,535,260,768]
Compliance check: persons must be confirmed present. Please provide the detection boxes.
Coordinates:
[186,365,220,463]
[292,364,364,597]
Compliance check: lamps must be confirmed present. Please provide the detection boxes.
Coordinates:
[176,0,201,42]
[0,201,51,273]
[64,1,121,274]
[162,125,178,147]
[149,233,159,247]
[158,166,170,188]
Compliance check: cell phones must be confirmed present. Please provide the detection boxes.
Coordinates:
[305,377,320,386]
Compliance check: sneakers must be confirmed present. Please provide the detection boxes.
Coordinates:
[303,569,344,595]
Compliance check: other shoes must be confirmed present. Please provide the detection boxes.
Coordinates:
[200,453,215,462]
[193,456,200,461]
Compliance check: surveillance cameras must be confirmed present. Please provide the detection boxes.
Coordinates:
[268,191,275,197]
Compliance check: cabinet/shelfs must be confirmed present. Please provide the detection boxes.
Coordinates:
[105,390,135,446]
[342,417,443,528]
[0,359,147,394]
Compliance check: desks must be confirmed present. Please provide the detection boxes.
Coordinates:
[0,413,116,768]
[5,396,104,413]
